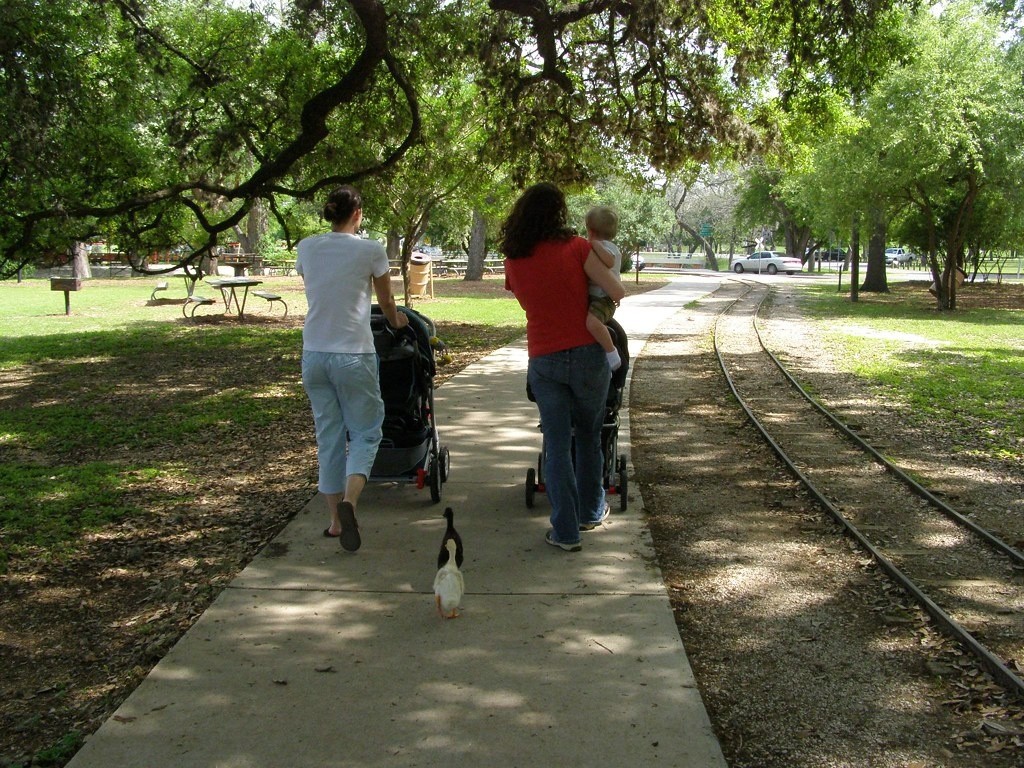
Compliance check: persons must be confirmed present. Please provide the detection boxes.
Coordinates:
[499,182,624,552]
[296,185,410,553]
[582,206,623,372]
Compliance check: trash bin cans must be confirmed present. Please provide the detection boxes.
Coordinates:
[409,253,432,296]
[929,266,968,301]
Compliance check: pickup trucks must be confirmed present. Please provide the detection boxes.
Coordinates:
[884,248,917,266]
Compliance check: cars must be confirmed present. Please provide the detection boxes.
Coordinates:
[630,255,646,271]
[730,250,803,275]
[805,247,848,262]
[410,244,443,257]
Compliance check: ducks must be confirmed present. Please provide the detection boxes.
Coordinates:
[431,507,465,619]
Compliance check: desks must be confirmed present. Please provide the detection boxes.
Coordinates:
[225,262,252,275]
[206,276,262,322]
[484,266,505,276]
[445,260,469,275]
[171,267,206,300]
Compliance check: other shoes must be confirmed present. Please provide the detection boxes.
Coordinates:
[337,501,361,551]
[324,526,339,537]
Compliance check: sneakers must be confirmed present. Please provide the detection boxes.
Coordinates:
[580,501,610,531]
[545,531,582,552]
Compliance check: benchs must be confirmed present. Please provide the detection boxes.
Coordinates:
[255,266,507,279]
[251,291,288,323]
[149,282,169,305]
[184,295,215,325]
[206,280,230,303]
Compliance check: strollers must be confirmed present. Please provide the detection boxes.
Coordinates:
[344,303,450,503]
[526,318,629,511]
